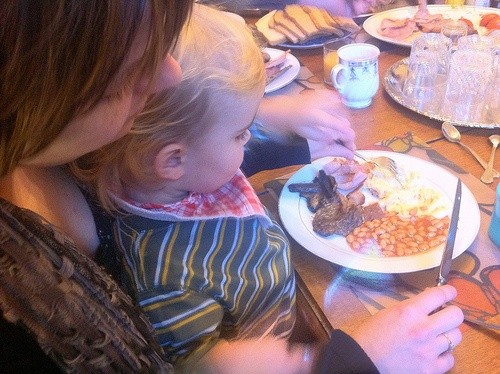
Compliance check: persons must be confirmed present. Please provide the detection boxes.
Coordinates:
[0,0,464,374]
[97,3,357,374]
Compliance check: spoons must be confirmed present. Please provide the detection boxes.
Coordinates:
[481,135,500,184]
[442,122,500,178]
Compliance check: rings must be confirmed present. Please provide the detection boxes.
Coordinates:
[441,332,455,353]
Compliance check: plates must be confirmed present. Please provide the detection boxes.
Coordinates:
[277,32,352,49]
[362,4,500,48]
[278,150,481,274]
[262,47,301,92]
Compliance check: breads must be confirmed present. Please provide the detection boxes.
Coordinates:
[254,4,358,45]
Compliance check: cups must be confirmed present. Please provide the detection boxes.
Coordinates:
[330,43,380,109]
[403,30,500,122]
[323,38,354,89]
[488,183,500,246]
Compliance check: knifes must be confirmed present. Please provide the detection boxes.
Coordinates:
[434,179,462,311]
